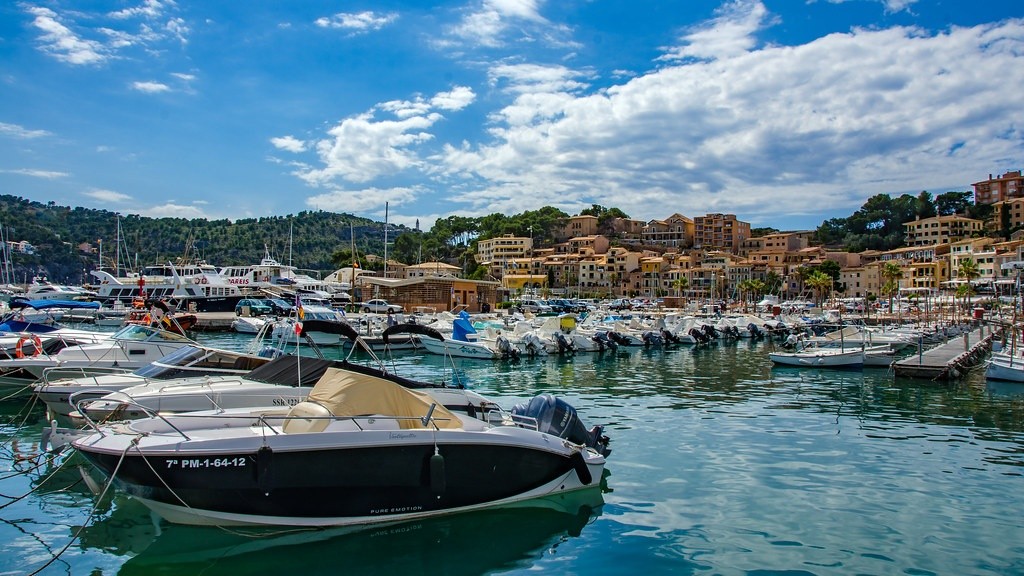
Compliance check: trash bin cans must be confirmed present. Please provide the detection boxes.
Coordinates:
[355,304,360,313]
[974,307,985,321]
[345,303,351,313]
[773,305,780,316]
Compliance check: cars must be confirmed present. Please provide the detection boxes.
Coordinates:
[519,299,600,314]
[362,298,404,313]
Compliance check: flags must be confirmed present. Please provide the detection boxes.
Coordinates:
[504,260,508,270]
[513,260,518,268]
[296,295,304,320]
[292,322,303,334]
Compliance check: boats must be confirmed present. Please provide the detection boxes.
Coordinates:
[1,314,612,530]
[614,305,763,349]
[1,214,355,313]
[980,294,1023,384]
[764,294,972,367]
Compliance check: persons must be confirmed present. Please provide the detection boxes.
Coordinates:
[720,303,727,314]
[380,311,393,334]
[495,330,509,351]
[858,303,862,316]
[907,300,920,312]
[482,303,490,313]
[764,305,771,313]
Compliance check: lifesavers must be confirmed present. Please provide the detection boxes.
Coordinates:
[201,278,207,284]
[195,277,200,284]
[16,335,43,358]
